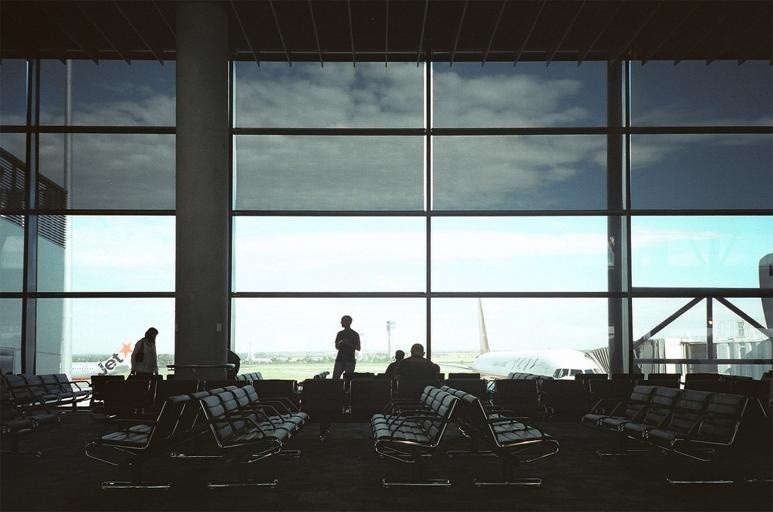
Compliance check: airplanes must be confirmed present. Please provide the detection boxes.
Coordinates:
[429,290,607,384]
[58,347,140,384]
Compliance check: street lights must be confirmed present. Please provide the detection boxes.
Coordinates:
[384,320,392,362]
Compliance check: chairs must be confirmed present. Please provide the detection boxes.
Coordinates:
[1,369,772,492]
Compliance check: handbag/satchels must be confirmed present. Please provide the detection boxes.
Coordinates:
[133,339,145,364]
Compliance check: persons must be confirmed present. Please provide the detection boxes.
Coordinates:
[333,315,361,414]
[227,349,241,380]
[395,343,436,396]
[385,350,405,375]
[131,327,158,416]
